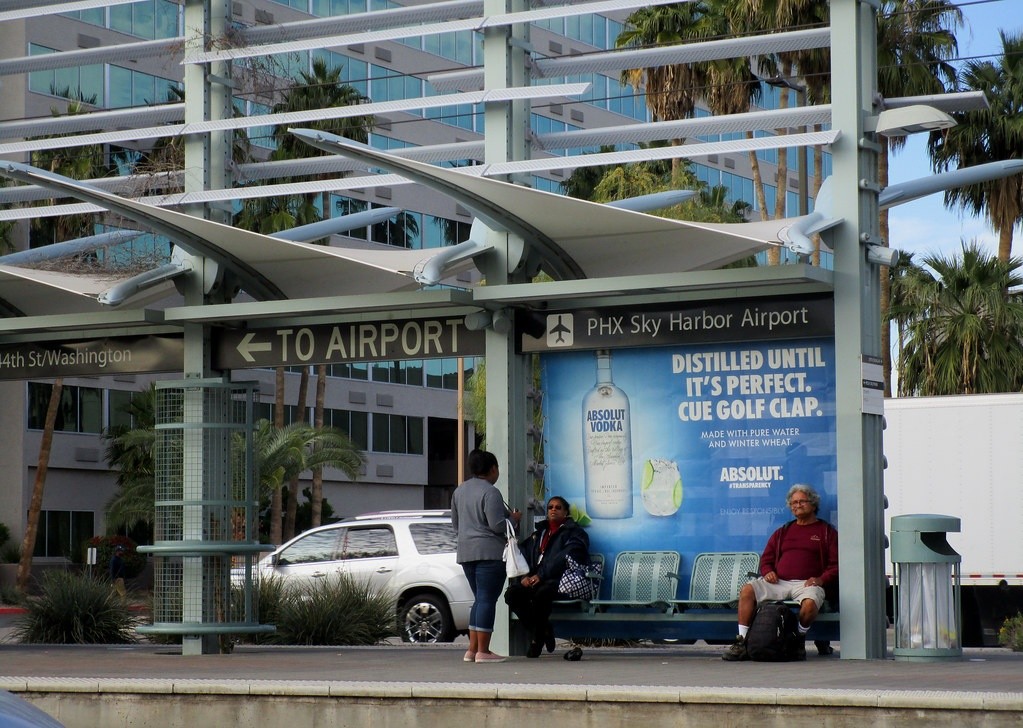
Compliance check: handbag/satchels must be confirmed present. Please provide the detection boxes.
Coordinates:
[557,555,602,601]
[501,519,530,578]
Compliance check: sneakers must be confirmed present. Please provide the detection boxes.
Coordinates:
[723,635,746,661]
[790,627,806,661]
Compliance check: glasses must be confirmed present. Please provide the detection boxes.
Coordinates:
[547,505,563,510]
[788,499,812,506]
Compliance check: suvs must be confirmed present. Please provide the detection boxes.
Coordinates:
[227,508,476,643]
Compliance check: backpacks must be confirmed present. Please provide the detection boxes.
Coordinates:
[744,600,797,662]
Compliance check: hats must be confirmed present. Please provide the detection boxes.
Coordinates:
[114,544,128,552]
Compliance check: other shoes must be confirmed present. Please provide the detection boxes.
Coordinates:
[526,632,547,658]
[463,651,476,661]
[475,651,507,663]
[814,639,833,654]
[544,626,555,653]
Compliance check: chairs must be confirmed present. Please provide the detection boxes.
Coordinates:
[590,550,681,616]
[769,600,801,605]
[553,552,605,616]
[669,551,760,616]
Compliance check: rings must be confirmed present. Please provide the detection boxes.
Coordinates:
[813,581,815,583]
[768,577,770,579]
[533,580,534,581]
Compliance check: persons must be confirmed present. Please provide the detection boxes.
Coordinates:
[451,449,522,662]
[504,496,589,658]
[721,484,839,661]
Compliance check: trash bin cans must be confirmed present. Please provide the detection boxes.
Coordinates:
[888,510,966,664]
